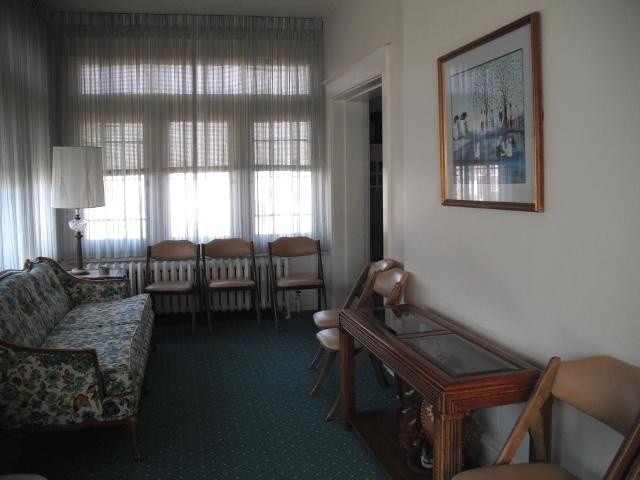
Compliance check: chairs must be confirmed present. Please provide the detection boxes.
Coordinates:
[201,238,265,330]
[266,237,329,332]
[308,260,395,390]
[454,354,639,478]
[311,267,411,421]
[147,239,203,330]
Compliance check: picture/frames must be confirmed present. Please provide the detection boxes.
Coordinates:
[435,12,545,213]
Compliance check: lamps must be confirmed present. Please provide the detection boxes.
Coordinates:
[50,145,108,274]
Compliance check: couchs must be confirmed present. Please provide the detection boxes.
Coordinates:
[1,257,154,462]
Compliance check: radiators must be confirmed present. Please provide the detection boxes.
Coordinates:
[89,256,289,315]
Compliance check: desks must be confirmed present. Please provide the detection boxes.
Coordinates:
[67,270,127,280]
[336,304,551,479]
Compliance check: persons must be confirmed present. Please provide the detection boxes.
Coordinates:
[453,103,520,159]
